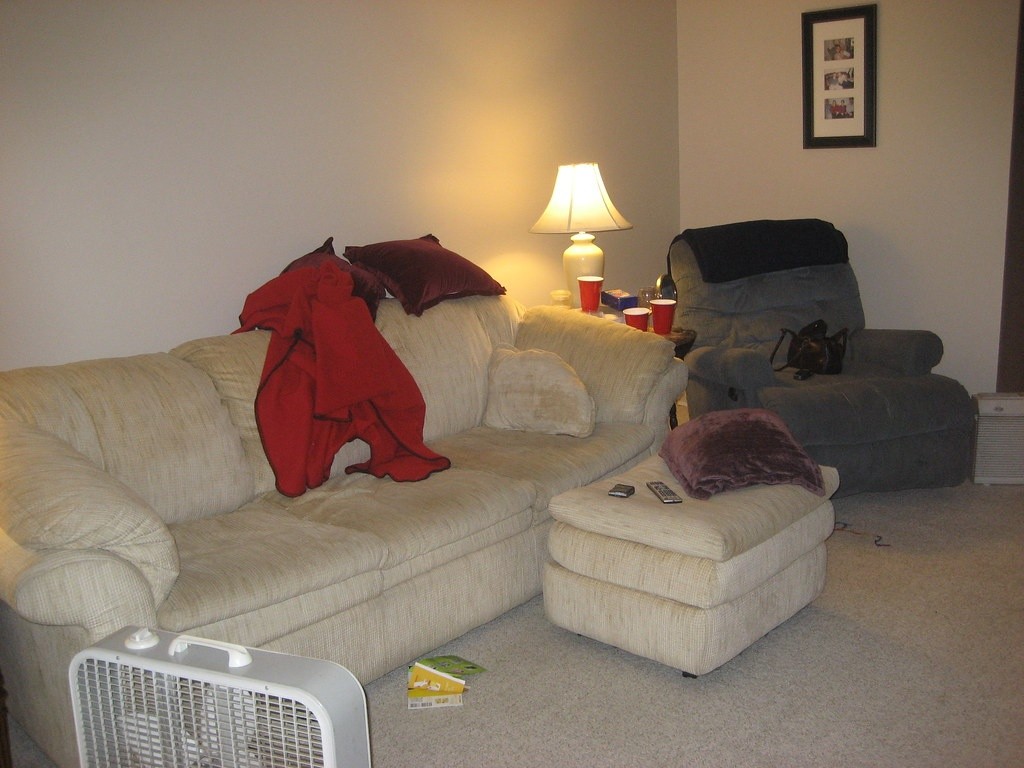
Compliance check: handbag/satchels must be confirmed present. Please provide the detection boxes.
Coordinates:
[770,320,848,380]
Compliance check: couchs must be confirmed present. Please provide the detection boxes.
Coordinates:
[0,295,690,768]
[666,218,978,501]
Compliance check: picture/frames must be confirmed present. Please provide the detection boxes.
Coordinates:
[800,3,877,149]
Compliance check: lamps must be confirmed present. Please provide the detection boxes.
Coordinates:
[531,162,633,310]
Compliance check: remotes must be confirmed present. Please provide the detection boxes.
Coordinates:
[646,481,683,504]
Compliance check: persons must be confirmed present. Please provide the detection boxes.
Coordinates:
[832,45,849,59]
[825,97,854,118]
[829,71,854,89]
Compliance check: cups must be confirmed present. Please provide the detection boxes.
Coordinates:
[576,276,604,314]
[638,287,662,310]
[549,290,571,312]
[621,308,651,332]
[650,300,678,335]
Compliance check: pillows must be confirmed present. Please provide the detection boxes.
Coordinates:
[659,408,829,506]
[481,343,596,437]
[277,234,507,329]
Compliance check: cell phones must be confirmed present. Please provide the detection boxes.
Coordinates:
[608,484,635,497]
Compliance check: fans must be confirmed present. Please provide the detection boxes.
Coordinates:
[68,624,371,768]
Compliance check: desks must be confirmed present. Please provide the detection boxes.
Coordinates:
[574,303,698,429]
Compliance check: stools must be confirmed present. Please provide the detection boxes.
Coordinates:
[540,444,842,677]
